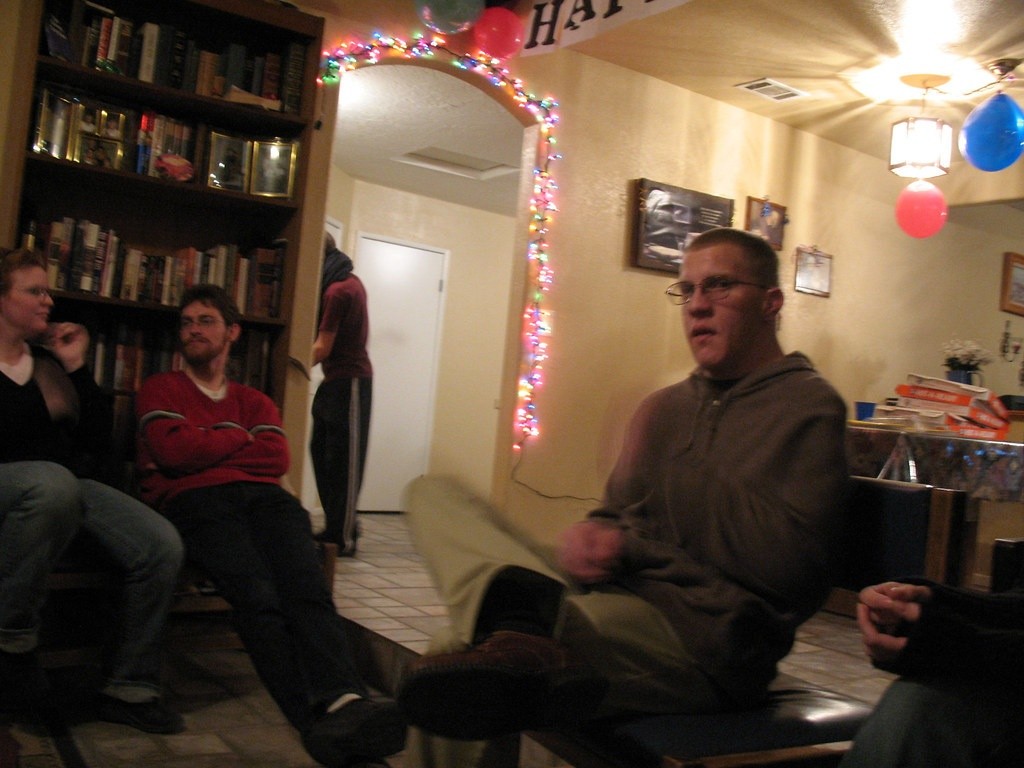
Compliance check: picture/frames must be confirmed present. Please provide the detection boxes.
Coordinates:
[1000,251,1024,316]
[746,197,788,251]
[794,247,833,297]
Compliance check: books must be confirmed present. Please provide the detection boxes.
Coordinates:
[895,181,948,238]
[19,216,287,320]
[84,332,268,393]
[958,94,1024,172]
[44,0,302,118]
[30,83,192,179]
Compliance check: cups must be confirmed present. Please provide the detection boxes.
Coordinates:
[854,401,876,421]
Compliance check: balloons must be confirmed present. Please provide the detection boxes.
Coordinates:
[474,7,523,59]
[416,0,484,34]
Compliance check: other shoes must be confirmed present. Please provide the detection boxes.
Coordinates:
[304,693,408,766]
[96,697,184,732]
[401,637,604,739]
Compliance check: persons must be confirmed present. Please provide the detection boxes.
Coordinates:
[133,284,411,768]
[842,578,1024,768]
[397,227,847,767]
[308,230,374,554]
[0,247,187,735]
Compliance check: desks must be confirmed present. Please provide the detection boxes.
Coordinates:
[845,425,1024,583]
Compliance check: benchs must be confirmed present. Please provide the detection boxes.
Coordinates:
[541,675,882,767]
[39,541,342,613]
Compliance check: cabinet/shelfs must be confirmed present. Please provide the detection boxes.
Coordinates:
[15,0,327,499]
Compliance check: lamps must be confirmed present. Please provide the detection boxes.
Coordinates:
[886,75,952,178]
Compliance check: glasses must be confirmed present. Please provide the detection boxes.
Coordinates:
[666,278,766,304]
[180,315,227,330]
[8,286,53,301]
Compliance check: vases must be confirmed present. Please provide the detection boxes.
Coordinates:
[947,369,973,384]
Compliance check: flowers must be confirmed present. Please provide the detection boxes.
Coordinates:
[943,336,988,372]
